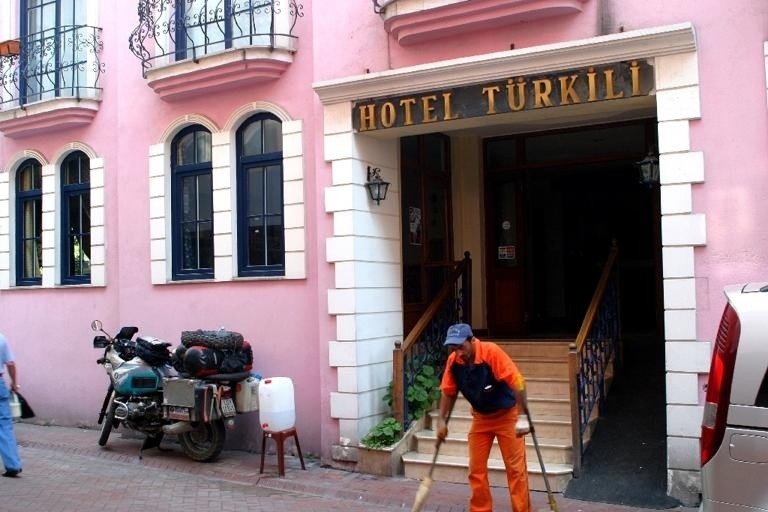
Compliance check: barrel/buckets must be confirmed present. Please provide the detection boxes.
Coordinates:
[258,377,296,433]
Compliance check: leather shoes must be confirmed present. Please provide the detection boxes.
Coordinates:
[4,468,22,476]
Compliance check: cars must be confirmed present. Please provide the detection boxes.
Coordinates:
[697,282,768,512]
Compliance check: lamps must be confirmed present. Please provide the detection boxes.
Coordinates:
[636,145,659,190]
[365,166,391,207]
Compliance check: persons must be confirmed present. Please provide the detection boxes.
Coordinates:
[0,330,22,477]
[437,324,531,512]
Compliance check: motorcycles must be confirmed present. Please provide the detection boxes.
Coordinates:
[90,320,258,462]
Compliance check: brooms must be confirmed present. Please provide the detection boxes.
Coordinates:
[411,390,459,512]
[527,411,558,512]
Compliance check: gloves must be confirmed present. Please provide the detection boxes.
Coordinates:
[515,415,530,438]
[438,423,448,442]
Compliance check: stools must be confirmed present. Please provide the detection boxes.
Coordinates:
[259,426,306,480]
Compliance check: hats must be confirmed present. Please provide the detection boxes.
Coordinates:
[443,323,473,346]
